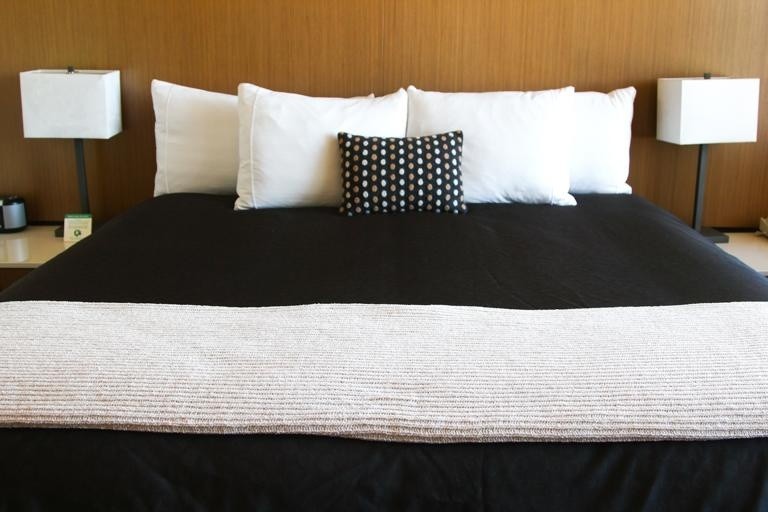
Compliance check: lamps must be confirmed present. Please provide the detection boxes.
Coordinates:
[655,73,760,243]
[19,64,123,237]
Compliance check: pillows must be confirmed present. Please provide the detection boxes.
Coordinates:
[569,86,637,195]
[337,130,468,216]
[151,78,375,200]
[234,83,408,213]
[407,84,577,207]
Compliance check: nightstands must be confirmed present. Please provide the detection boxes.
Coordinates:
[1,225,79,297]
[0,195,767,511]
[715,231,768,279]
